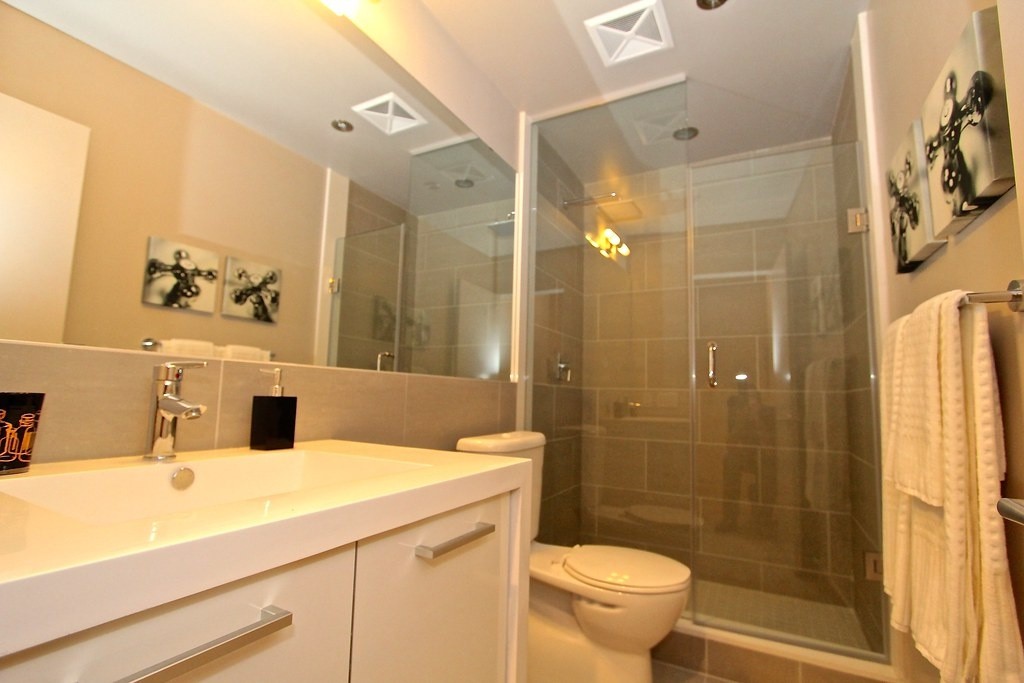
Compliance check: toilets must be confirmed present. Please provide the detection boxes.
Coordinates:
[456,425,694,683]
[578,422,710,569]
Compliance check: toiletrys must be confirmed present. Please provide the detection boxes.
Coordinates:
[248,366,300,453]
[0,388,47,478]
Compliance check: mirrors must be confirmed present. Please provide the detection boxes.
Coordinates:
[338,181,514,383]
[1,0,330,368]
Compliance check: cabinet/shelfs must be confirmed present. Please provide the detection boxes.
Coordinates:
[349,491,509,683]
[0,540,357,683]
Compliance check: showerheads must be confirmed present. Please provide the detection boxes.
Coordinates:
[486,216,514,239]
[596,197,646,226]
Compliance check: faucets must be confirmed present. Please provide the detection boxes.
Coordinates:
[555,362,575,385]
[142,360,211,464]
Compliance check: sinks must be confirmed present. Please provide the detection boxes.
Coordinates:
[0,440,469,543]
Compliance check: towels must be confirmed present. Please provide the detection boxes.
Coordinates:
[894,289,1008,511]
[881,309,923,635]
[799,356,860,617]
[904,290,1024,683]
[159,336,277,364]
